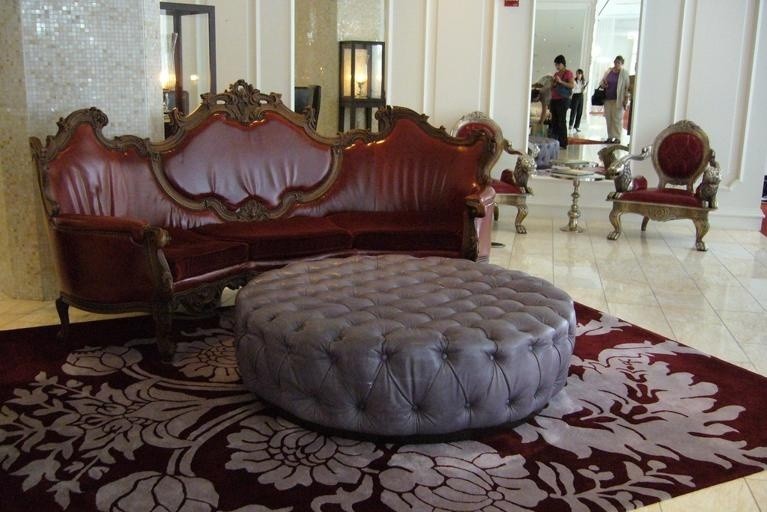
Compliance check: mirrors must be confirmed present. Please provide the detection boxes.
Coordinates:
[160,1,218,130]
[524,0,647,181]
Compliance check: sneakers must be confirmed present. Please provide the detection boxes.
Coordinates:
[568,124,582,133]
[604,138,621,144]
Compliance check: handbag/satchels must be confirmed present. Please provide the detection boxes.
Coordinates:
[592,85,607,106]
[556,87,573,99]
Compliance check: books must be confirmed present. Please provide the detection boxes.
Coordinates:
[564,159,590,164]
[551,167,595,177]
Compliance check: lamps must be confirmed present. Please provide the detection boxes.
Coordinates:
[160,32,179,112]
[338,41,384,131]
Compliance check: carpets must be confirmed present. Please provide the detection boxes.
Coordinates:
[0,301,767,512]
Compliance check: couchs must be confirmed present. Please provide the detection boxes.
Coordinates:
[27,79,504,361]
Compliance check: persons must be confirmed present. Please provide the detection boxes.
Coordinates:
[569,69,589,132]
[598,55,630,144]
[548,54,575,151]
[627,62,637,135]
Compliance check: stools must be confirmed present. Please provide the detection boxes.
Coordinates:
[529,135,560,169]
[232,254,578,439]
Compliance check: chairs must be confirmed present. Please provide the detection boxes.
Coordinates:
[448,109,537,234]
[597,145,629,169]
[605,119,721,251]
